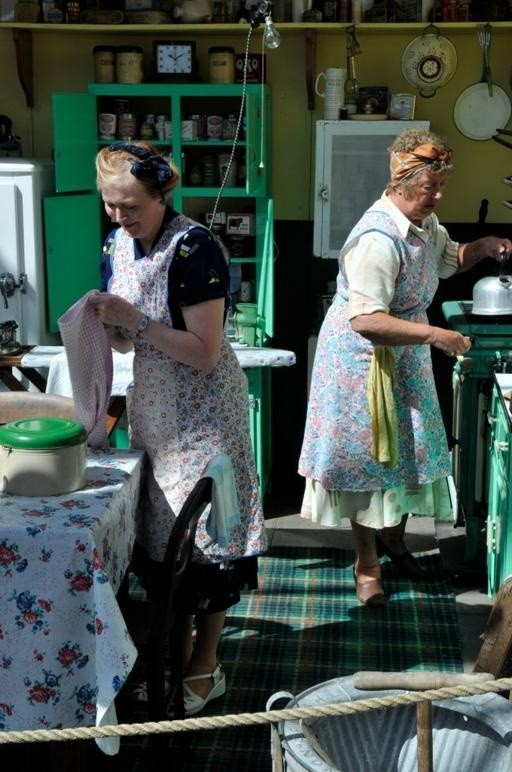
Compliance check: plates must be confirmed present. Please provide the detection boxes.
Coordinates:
[349,112,388,121]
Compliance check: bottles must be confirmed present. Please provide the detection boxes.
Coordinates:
[234,304,264,348]
[120,111,166,142]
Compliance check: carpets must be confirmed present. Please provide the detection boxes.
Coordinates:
[86,545,462,772]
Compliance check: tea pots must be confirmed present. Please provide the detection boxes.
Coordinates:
[472,245,512,315]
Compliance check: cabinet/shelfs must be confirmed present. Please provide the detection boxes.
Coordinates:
[485,386,511,597]
[313,119,429,261]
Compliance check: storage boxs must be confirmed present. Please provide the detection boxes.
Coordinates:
[43,191,272,347]
[51,80,270,198]
[115,361,270,501]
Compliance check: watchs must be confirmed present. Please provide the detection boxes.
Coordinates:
[138,316,150,336]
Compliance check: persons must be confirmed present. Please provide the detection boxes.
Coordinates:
[89,141,268,717]
[296,129,512,607]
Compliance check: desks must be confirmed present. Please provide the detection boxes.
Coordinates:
[1,345,297,439]
[1,446,148,757]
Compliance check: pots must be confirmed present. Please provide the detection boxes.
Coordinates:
[1,414,87,494]
[401,23,511,141]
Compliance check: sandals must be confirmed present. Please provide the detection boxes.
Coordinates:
[132,668,177,704]
[166,660,226,718]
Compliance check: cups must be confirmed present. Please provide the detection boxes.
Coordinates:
[315,68,347,120]
[219,153,236,185]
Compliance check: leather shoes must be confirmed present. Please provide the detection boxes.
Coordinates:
[353,561,386,611]
[376,535,428,580]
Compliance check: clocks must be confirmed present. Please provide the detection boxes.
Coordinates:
[153,40,197,81]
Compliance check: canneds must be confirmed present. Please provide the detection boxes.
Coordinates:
[200,155,218,186]
[241,280,251,302]
